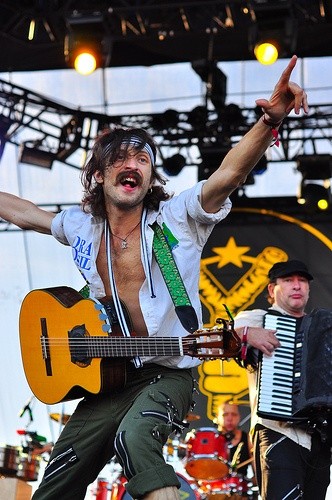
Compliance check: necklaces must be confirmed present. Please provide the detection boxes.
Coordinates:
[110,220,141,249]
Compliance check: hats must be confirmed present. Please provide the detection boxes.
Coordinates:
[268,260,313,282]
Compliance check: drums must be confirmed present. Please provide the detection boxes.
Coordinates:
[96,477,127,500]
[0,444,38,481]
[118,472,209,500]
[190,472,257,500]
[184,427,232,480]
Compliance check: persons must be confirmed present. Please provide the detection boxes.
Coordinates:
[0,54,310,500]
[217,404,255,482]
[204,259,332,500]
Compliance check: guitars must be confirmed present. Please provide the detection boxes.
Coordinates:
[18,287,242,405]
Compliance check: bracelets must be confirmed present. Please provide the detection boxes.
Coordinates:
[262,114,284,148]
[241,326,248,360]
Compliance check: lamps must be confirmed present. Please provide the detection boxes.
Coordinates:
[64,31,107,74]
[16,139,54,170]
[163,153,187,176]
[251,28,281,65]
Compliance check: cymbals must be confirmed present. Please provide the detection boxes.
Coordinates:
[50,413,71,424]
[184,413,200,422]
